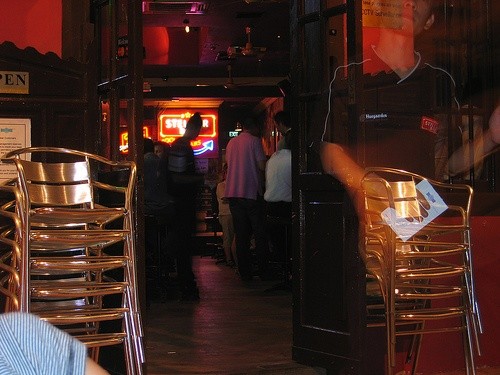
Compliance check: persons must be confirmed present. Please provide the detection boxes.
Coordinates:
[318,2,499,340]
[141,108,299,304]
[0,309,110,375]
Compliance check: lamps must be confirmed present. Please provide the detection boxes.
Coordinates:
[238,24,258,58]
[219,57,235,89]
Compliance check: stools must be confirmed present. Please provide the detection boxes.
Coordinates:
[263,215,289,282]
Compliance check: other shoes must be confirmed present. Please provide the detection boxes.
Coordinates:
[239,267,252,281]
[167,275,182,299]
[226,258,240,267]
[180,281,199,302]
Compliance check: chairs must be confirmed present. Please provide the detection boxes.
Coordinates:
[0,146,145,375]
[358,166,483,375]
